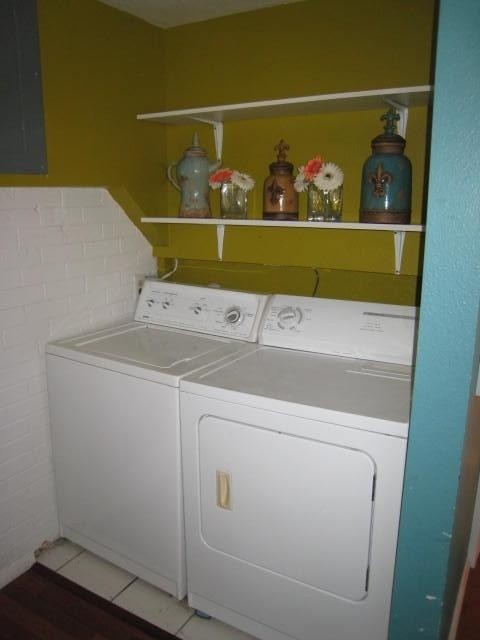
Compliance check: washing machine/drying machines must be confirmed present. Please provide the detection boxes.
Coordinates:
[177,294,420,640]
[45,277,270,601]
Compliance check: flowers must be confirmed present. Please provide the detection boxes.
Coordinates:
[207,167,256,216]
[293,156,344,219]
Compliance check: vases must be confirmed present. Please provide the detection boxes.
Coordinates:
[219,184,247,220]
[305,181,343,223]
